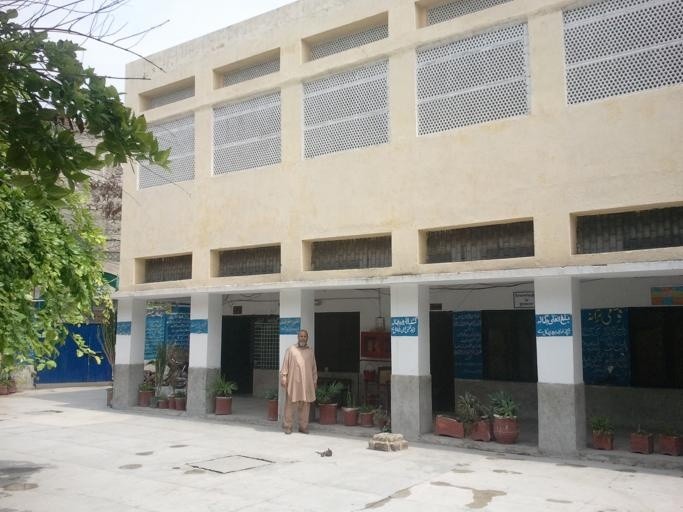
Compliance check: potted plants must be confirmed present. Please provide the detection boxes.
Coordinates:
[629,423,681,457]
[135,383,184,410]
[205,373,239,414]
[434,389,522,443]
[263,388,277,420]
[312,379,389,431]
[590,416,617,450]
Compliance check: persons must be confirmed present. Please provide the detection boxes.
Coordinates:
[279,330,318,434]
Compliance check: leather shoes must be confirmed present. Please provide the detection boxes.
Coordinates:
[285,429,291,434]
[298,428,308,433]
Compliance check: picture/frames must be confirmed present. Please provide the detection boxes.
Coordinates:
[358,330,392,363]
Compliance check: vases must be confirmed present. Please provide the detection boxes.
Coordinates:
[0,379,15,394]
